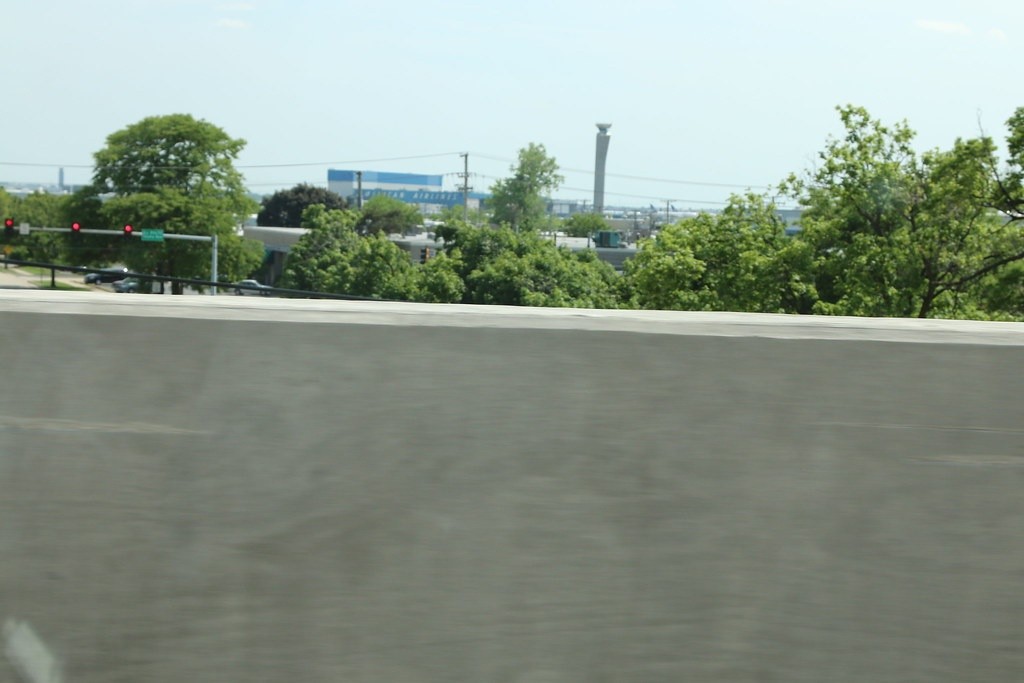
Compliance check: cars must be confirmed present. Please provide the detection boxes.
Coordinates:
[227,280,274,295]
[112,276,161,295]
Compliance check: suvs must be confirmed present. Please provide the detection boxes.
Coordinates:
[84,266,129,285]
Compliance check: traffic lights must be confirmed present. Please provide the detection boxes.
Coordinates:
[419,247,430,264]
[5,217,15,238]
[71,222,80,241]
[123,224,133,242]
